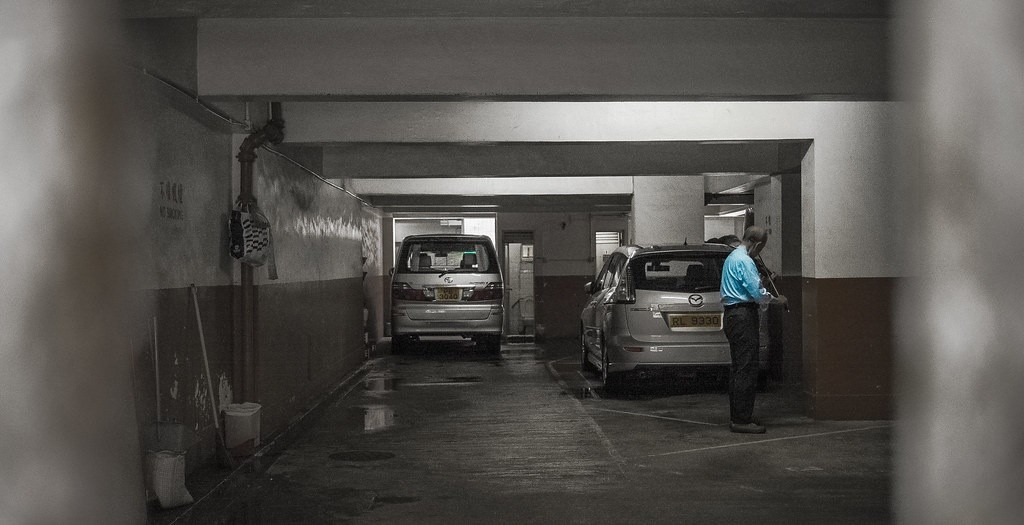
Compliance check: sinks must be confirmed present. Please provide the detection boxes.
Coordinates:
[523,318,534,327]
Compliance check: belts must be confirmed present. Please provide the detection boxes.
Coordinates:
[725,302,757,310]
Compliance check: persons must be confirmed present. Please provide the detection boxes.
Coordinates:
[719,223,789,432]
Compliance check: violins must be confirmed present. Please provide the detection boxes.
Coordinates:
[750,256,772,278]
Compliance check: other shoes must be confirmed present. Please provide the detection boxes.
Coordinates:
[730,423,766,433]
[729,420,760,427]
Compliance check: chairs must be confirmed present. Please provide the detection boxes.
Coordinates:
[630,268,648,290]
[683,265,707,293]
[455,253,478,273]
[416,253,434,273]
